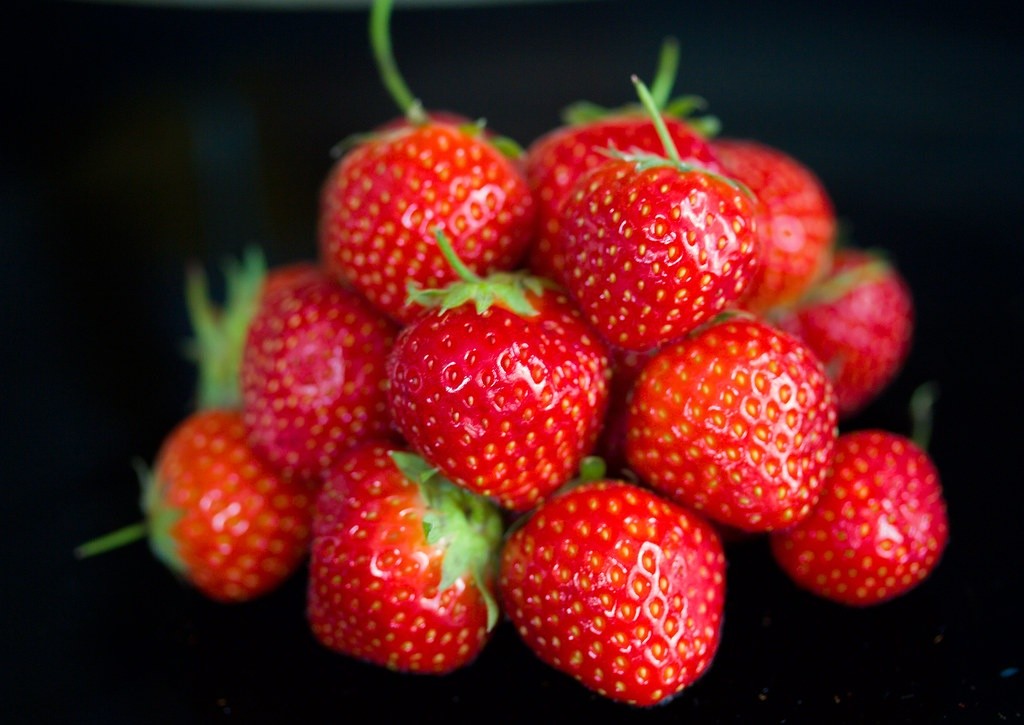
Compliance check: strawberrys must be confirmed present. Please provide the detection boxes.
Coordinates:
[72,0,945,708]
